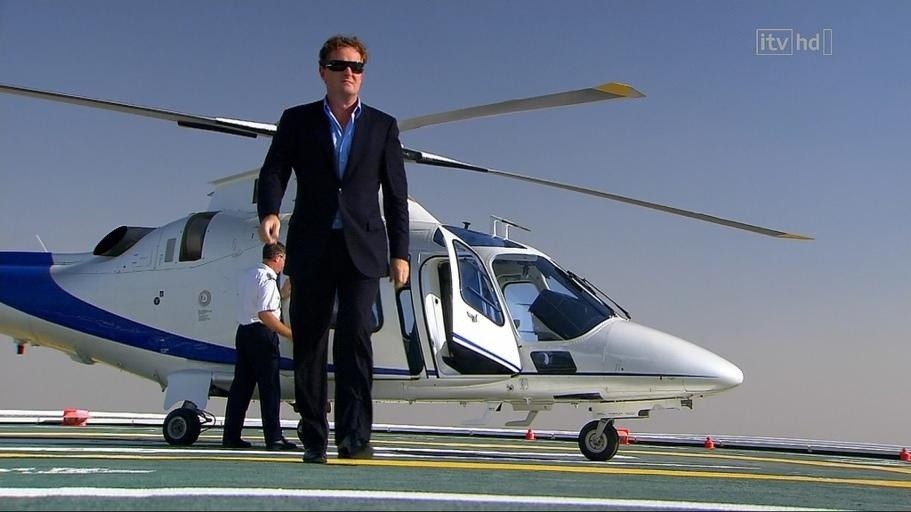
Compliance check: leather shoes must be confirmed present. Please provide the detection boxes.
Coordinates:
[303,449,327,462]
[224,440,251,448]
[266,437,296,451]
[338,441,373,459]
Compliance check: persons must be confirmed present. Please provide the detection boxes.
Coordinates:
[257,34,410,462]
[222,242,298,451]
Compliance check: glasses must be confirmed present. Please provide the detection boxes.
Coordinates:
[323,60,364,73]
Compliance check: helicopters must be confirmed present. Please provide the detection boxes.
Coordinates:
[0,77,813,464]
